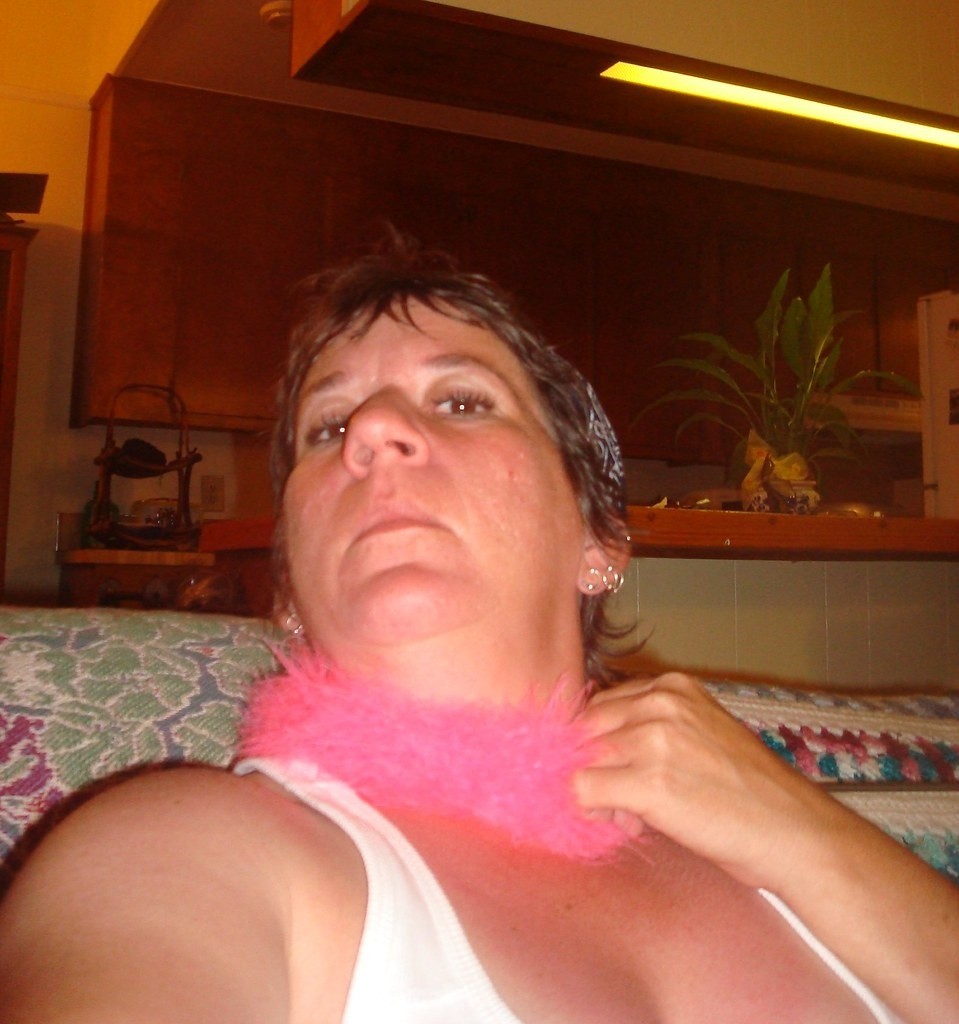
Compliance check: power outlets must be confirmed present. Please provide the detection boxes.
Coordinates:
[199,473,226,512]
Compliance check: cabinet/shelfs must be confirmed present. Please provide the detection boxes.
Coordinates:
[64,68,959,474]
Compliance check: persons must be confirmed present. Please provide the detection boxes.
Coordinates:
[0,234,958,1024]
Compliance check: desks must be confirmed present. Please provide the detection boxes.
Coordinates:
[58,546,218,610]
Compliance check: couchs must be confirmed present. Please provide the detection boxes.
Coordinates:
[0,603,959,888]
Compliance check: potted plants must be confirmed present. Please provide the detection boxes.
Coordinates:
[630,260,925,508]
